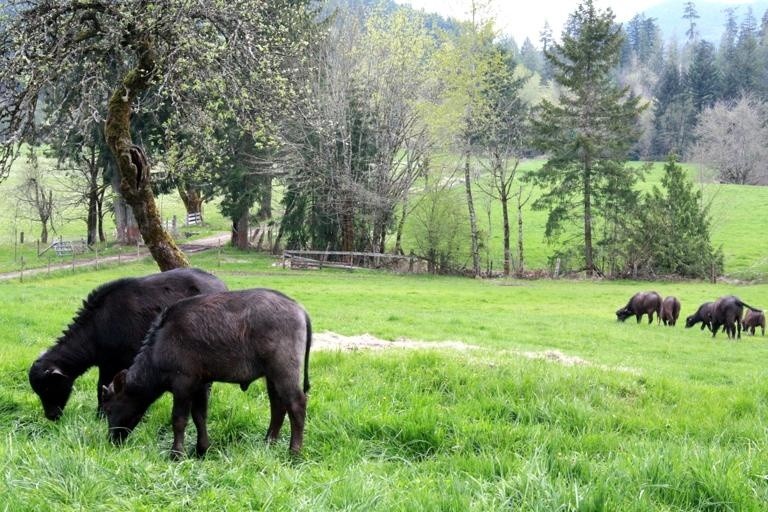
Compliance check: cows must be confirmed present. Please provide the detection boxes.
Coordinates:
[741,305,765,337]
[615,290,663,325]
[27,265,230,422]
[685,300,716,330]
[98,287,313,462]
[658,295,682,326]
[707,295,763,340]
